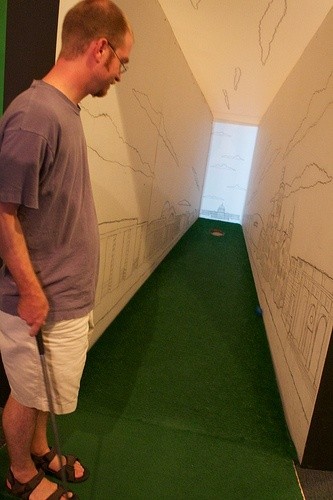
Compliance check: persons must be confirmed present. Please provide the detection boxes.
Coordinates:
[0,0,132,499]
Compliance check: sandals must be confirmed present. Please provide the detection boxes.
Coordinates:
[6,465,80,500]
[31,445,91,483]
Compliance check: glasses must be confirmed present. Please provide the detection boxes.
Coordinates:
[108,41,129,73]
